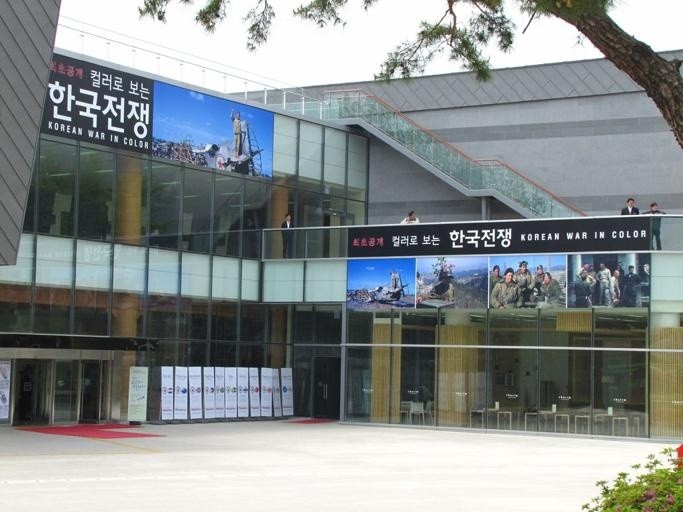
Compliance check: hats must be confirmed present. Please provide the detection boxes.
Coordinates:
[492,261,552,281]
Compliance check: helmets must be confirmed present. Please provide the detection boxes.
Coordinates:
[439,269,454,280]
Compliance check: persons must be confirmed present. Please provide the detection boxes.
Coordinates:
[374,287,385,309]
[245,217,260,257]
[399,211,420,224]
[511,260,531,294]
[539,272,562,302]
[488,268,523,309]
[280,213,294,258]
[620,197,640,217]
[533,263,546,294]
[489,265,501,291]
[387,285,401,304]
[575,258,650,307]
[230,107,241,155]
[642,203,668,250]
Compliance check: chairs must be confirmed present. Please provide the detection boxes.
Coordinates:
[399,399,435,426]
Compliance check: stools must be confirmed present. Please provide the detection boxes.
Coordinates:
[524,412,628,437]
[469,409,512,429]
